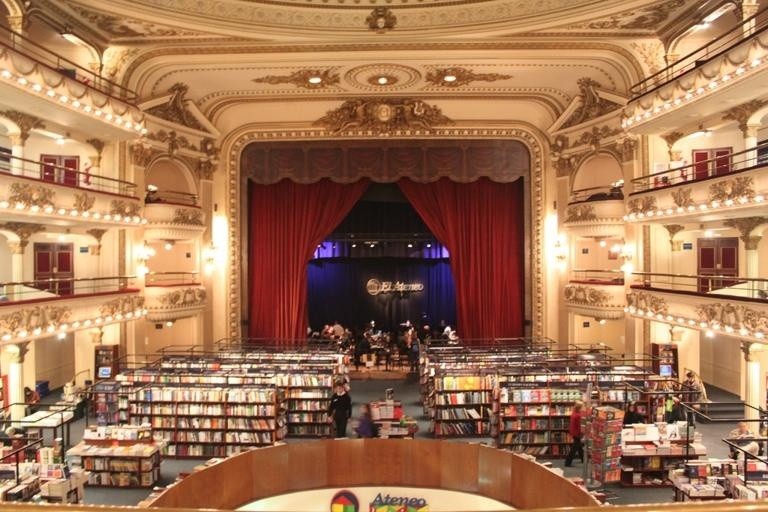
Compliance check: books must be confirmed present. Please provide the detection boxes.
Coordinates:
[66,425,169,487]
[669,458,768,505]
[368,389,419,439]
[1,439,89,506]
[421,347,645,456]
[585,409,703,486]
[93,351,351,456]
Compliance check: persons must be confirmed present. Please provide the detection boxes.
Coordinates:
[297,314,462,370]
[676,371,705,430]
[24,387,41,416]
[354,403,374,439]
[144,184,158,203]
[729,421,760,459]
[326,382,353,438]
[564,403,585,467]
[2,427,27,446]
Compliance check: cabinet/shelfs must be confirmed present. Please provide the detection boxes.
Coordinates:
[0,336,349,503]
[417,336,768,504]
[364,399,420,439]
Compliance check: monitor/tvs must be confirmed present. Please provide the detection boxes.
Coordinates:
[660,363,673,377]
[96,365,112,379]
